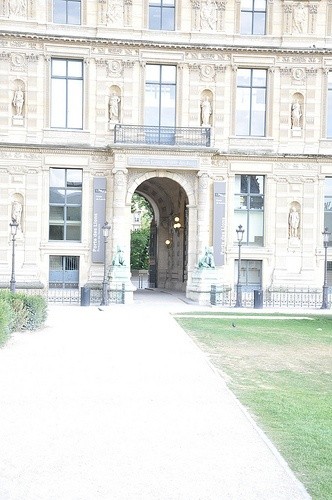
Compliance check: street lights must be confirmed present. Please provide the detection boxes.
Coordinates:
[321,226,331,309]
[9,220,19,291]
[102,221,111,304]
[236,224,244,307]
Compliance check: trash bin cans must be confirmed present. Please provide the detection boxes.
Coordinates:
[254,289,263,309]
[81,286,90,305]
